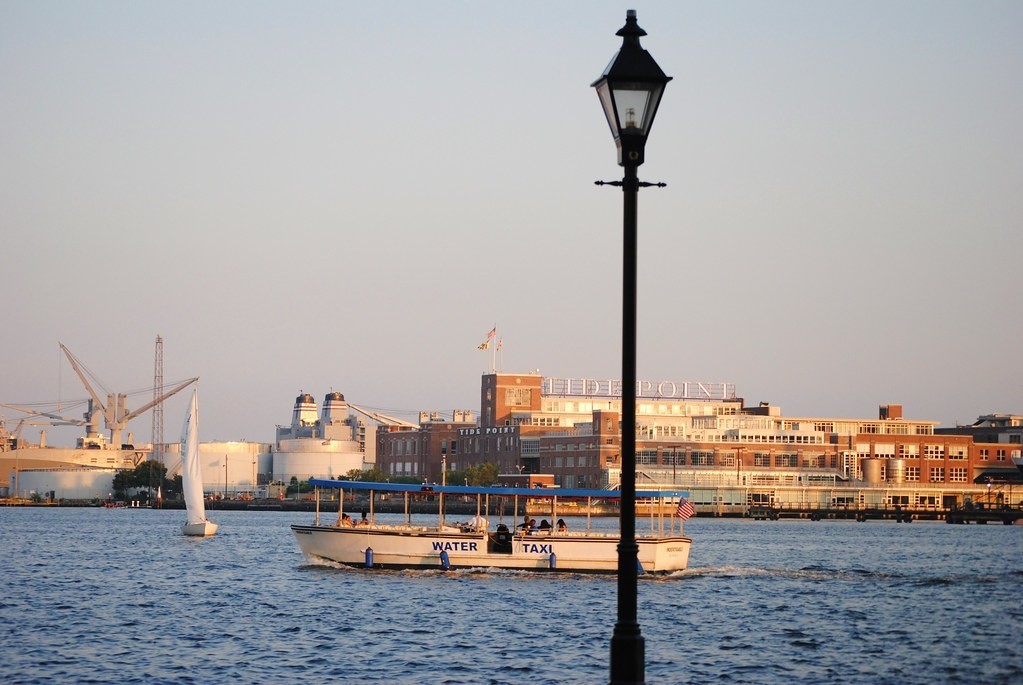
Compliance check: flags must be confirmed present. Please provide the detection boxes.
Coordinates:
[675,498,697,521]
[477,328,503,352]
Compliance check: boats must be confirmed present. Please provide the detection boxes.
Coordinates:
[291,478,693,572]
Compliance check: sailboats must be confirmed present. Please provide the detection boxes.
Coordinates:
[180,388,220,537]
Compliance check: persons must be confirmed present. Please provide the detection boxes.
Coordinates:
[517,515,567,535]
[457,512,489,533]
[334,511,369,528]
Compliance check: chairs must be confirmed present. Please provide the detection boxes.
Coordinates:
[530,531,657,538]
[355,524,429,532]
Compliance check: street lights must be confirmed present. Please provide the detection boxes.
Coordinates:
[278,479,281,497]
[349,478,353,499]
[987,483,991,509]
[386,478,390,484]
[424,478,428,503]
[593,11,677,685]
[464,478,468,504]
[732,447,747,483]
[666,445,682,482]
